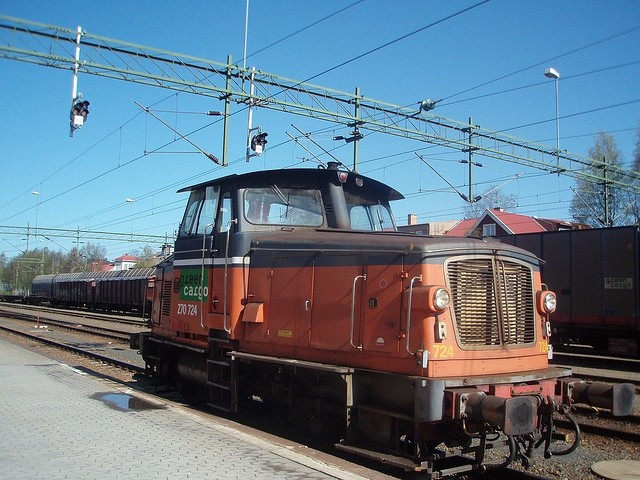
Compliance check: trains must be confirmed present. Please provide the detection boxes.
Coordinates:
[130,161,580,479]
[32,225,640,360]
